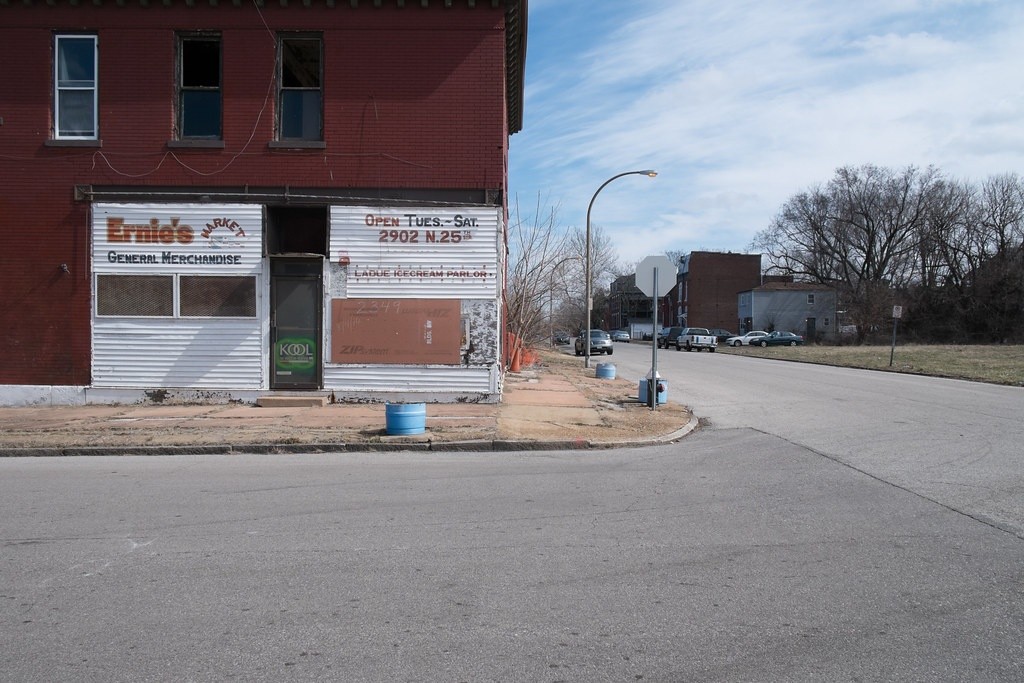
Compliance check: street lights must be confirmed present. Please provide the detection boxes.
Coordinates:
[584,168,658,369]
[548,256,580,348]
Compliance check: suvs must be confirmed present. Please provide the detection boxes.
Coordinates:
[658,326,684,348]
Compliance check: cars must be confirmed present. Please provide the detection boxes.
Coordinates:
[750,330,803,348]
[553,331,570,345]
[574,329,613,357]
[726,331,769,347]
[613,330,630,343]
[709,329,737,342]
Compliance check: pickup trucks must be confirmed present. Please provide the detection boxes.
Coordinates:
[676,328,718,352]
